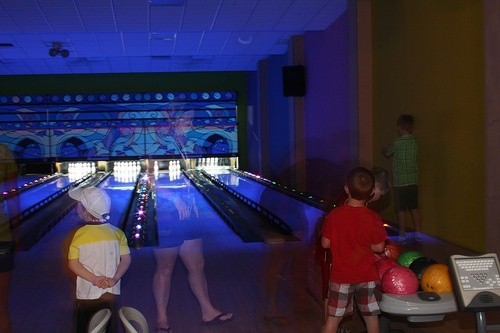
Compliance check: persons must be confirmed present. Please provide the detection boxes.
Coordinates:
[68,187,131,333]
[385,114,425,246]
[149,107,234,333]
[260,160,391,326]
[321,166,388,333]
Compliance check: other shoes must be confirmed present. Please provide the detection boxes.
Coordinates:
[414,234,423,243]
[395,234,408,246]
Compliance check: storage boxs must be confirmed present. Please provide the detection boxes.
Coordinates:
[377,290,459,322]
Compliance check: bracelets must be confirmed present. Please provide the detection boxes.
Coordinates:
[93,276,97,286]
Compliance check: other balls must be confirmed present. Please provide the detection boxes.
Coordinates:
[375,236,456,295]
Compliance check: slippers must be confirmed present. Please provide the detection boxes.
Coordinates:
[159,326,173,333]
[202,312,235,324]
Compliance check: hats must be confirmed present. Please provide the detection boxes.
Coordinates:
[67,186,111,222]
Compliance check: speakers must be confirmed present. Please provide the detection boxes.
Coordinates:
[283,65,306,96]
